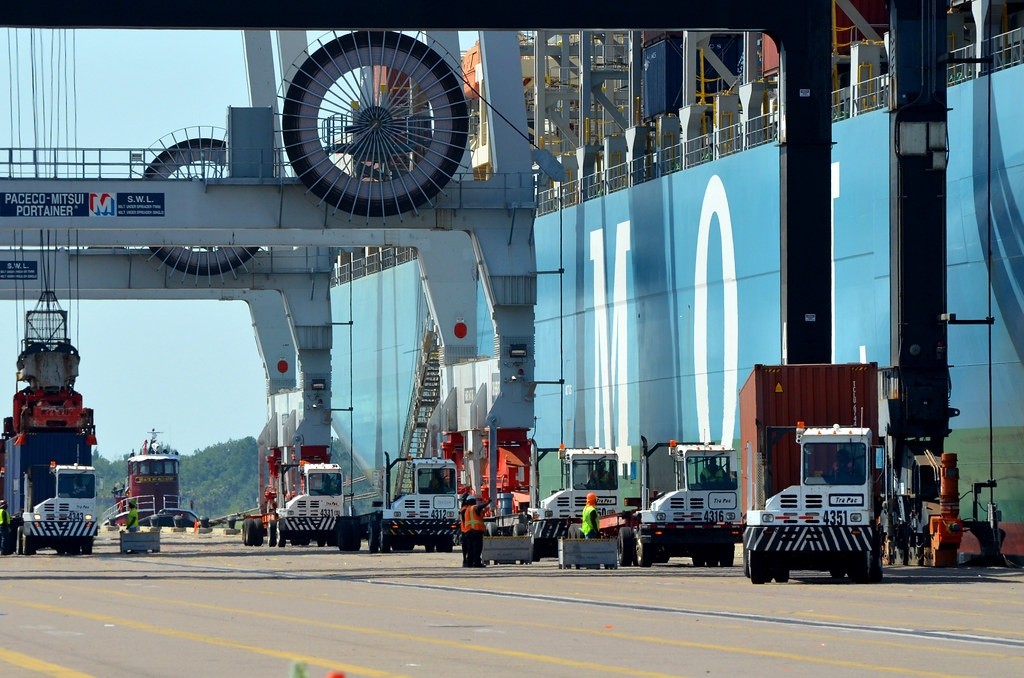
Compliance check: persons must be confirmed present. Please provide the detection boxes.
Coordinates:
[429,473,448,487]
[126,499,139,554]
[700,458,729,489]
[322,474,337,491]
[73,478,86,492]
[0,500,13,554]
[460,494,492,567]
[590,462,611,490]
[580,493,601,539]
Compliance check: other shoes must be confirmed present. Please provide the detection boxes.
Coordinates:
[472,562,486,568]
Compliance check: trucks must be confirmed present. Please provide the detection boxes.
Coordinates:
[369,457,459,552]
[599,436,741,567]
[737,361,886,583]
[240,463,362,552]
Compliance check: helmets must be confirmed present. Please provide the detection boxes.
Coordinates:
[0,500,7,508]
[467,495,476,500]
[462,493,470,499]
[129,499,137,506]
[586,493,597,501]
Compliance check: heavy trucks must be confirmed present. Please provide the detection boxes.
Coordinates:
[1,431,99,554]
[483,447,618,541]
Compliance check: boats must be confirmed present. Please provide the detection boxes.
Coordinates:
[113,457,180,526]
